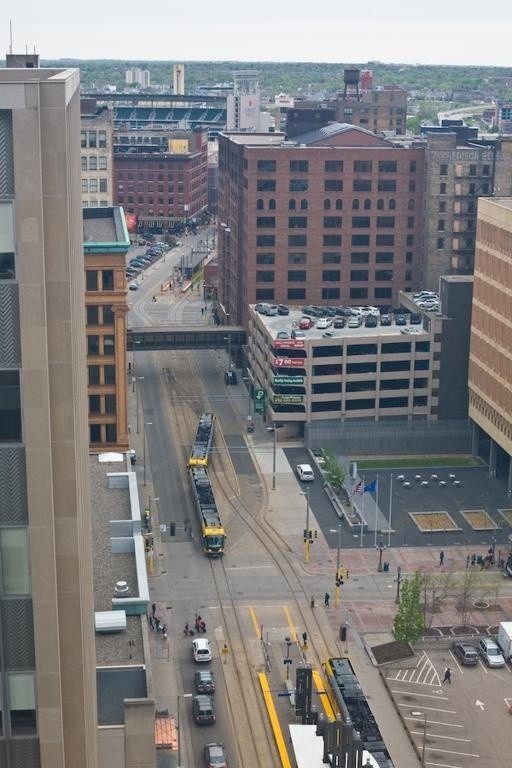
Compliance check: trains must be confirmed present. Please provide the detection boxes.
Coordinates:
[322,657,395,768]
[185,466,228,558]
[189,413,216,466]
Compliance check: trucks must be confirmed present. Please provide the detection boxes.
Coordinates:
[497,622,512,664]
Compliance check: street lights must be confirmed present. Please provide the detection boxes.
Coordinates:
[492,536,496,566]
[132,340,141,392]
[412,709,428,768]
[148,495,160,510]
[136,374,144,434]
[266,422,276,491]
[330,524,341,608]
[143,422,152,486]
[242,375,253,416]
[177,694,194,766]
[225,332,231,372]
[376,540,386,571]
[394,568,403,604]
[299,486,310,563]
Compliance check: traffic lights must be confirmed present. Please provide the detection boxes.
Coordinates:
[336,567,350,581]
[304,529,318,539]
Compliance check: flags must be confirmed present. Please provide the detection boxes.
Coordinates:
[348,481,363,499]
[364,480,377,494]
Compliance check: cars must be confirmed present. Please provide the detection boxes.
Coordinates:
[204,742,227,768]
[451,641,478,666]
[195,671,215,694]
[298,305,422,329]
[401,327,417,334]
[255,303,289,316]
[413,290,440,311]
[291,329,306,339]
[129,284,136,290]
[191,694,216,726]
[278,331,289,339]
[479,638,505,668]
[125,240,168,278]
[296,463,315,482]
[322,332,335,338]
[192,638,212,662]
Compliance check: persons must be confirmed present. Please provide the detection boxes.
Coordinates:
[438,550,494,570]
[149,604,206,637]
[442,668,451,684]
[324,592,330,608]
[310,595,315,608]
